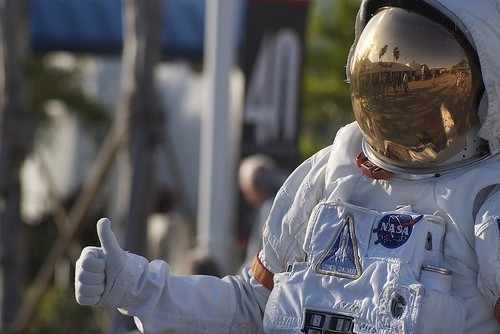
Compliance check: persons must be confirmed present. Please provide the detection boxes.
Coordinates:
[378,68,464,98]
[146,183,194,275]
[238,153,281,268]
[18,90,97,278]
[75,0,500,334]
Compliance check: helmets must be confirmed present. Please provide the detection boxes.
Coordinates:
[346,0,500,156]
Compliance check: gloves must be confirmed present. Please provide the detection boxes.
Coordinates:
[75,217,125,307]
